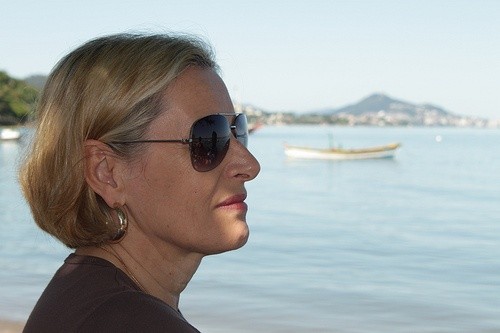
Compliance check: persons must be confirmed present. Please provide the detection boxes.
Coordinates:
[19,33,261,333]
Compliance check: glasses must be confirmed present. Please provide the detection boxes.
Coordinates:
[96,112,249,172]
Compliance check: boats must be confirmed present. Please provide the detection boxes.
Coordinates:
[283,141,399,159]
